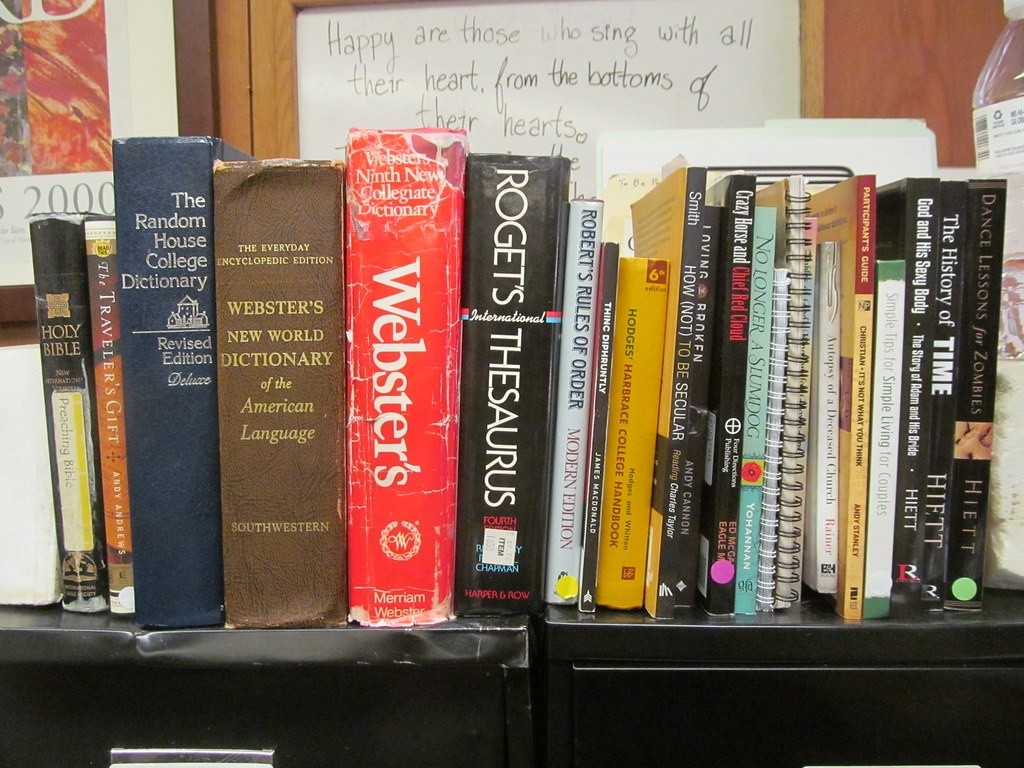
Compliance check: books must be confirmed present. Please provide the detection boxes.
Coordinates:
[213,155,350,629]
[346,126,471,626]
[27,210,109,614]
[456,148,572,617]
[113,134,257,628]
[81,215,135,617]
[545,167,1023,621]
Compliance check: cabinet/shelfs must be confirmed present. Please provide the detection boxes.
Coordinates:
[541,586,1024,768]
[0,599,537,768]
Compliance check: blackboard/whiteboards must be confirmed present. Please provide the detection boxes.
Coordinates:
[292,0,805,198]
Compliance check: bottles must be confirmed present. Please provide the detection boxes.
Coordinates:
[971,1,1024,361]
